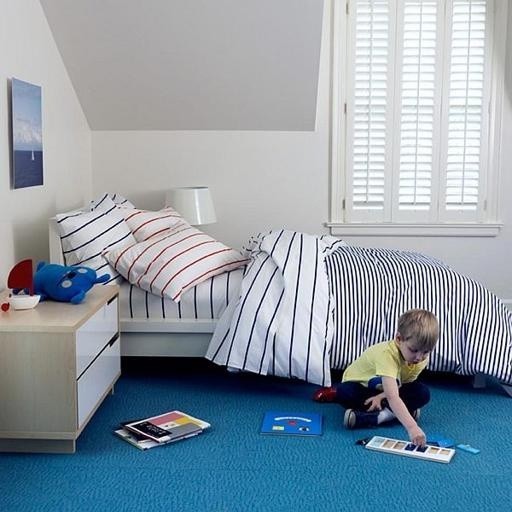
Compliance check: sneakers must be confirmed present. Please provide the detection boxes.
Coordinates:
[342,407,380,430]
[411,408,421,421]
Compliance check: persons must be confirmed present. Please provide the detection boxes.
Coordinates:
[312,309,439,451]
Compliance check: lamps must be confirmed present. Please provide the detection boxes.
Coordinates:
[164,186,219,226]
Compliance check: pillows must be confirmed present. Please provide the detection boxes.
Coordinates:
[54,192,250,305]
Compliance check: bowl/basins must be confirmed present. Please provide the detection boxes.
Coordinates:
[7,294,41,311]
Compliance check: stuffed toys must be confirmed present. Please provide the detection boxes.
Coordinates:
[10,258,111,304]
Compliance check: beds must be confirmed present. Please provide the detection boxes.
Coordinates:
[47,206,489,389]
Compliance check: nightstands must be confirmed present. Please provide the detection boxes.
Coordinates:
[1,284,123,458]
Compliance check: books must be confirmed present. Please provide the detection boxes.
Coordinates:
[113,425,204,452]
[260,408,323,439]
[125,409,212,444]
[120,417,203,442]
